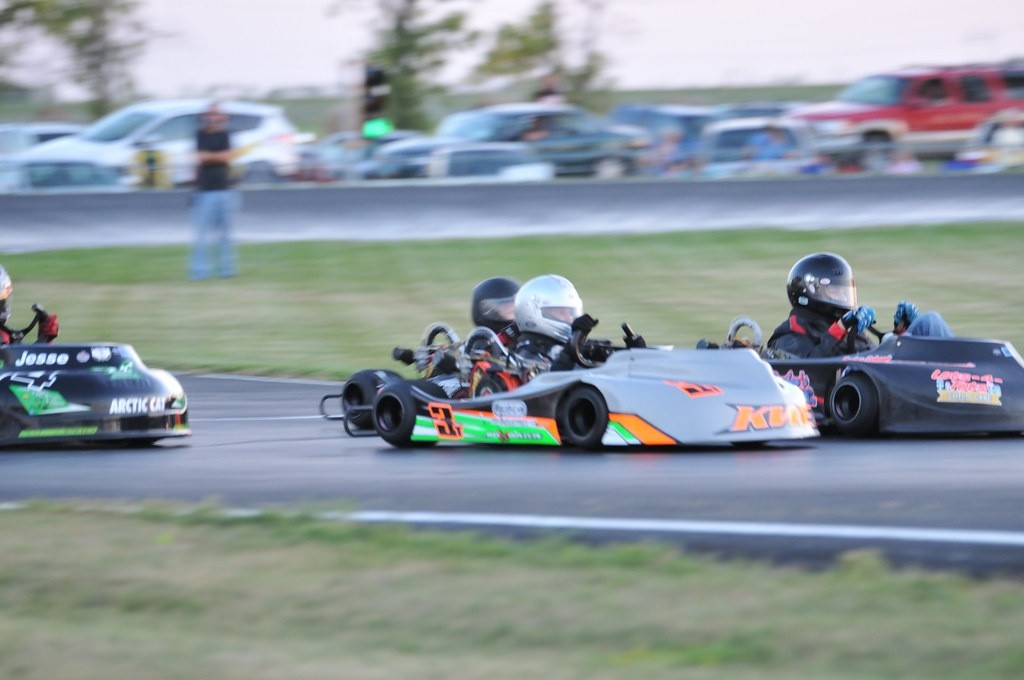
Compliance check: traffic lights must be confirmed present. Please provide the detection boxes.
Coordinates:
[362,64,395,142]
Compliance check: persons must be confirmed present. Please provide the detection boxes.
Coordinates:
[462,275,647,383]
[766,252,955,359]
[533,72,566,107]
[0,266,60,345]
[190,106,239,277]
[518,118,576,142]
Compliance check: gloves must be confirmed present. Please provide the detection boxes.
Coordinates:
[0,328,10,344]
[623,334,645,349]
[892,301,920,334]
[841,305,875,334]
[571,314,599,344]
[38,312,58,343]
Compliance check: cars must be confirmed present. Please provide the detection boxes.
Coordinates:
[0,58,1024,191]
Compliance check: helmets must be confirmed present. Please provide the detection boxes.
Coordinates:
[0,266,12,327]
[514,275,583,344]
[786,252,856,322]
[472,277,519,333]
